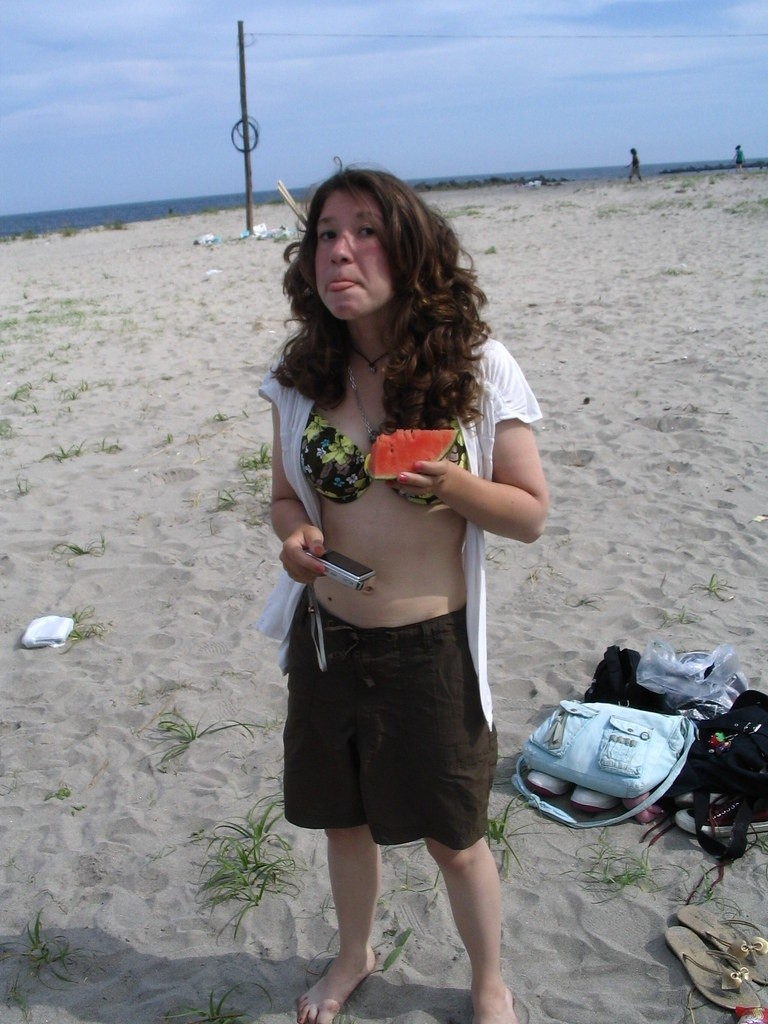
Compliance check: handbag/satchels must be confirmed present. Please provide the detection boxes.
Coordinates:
[510,698,693,828]
[636,638,751,719]
[663,690,768,862]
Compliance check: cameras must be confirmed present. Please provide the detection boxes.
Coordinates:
[303,549,376,590]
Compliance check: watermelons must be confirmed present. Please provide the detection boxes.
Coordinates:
[366,428,460,479]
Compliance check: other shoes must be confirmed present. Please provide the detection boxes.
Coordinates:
[525,768,574,796]
[569,784,621,813]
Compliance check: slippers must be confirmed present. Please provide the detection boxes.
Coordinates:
[663,926,763,1011]
[676,906,768,985]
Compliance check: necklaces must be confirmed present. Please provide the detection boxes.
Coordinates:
[354,348,389,373]
[347,365,380,444]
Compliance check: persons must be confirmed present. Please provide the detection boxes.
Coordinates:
[254,168,549,1024]
[625,148,642,182]
[733,145,746,171]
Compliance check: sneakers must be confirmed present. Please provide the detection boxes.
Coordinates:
[675,794,768,837]
